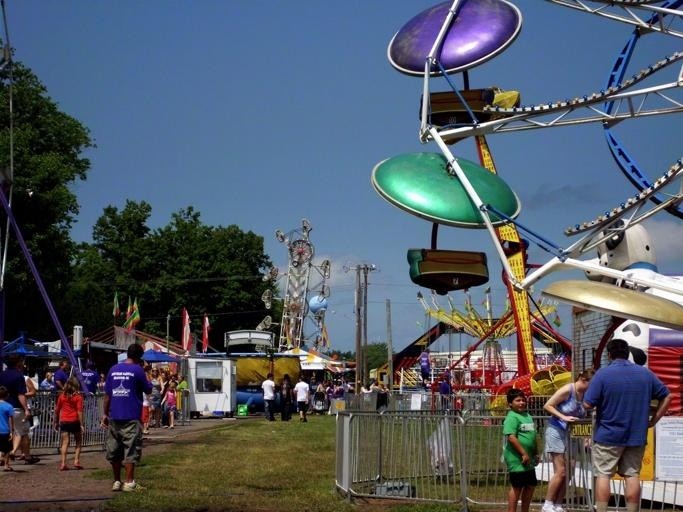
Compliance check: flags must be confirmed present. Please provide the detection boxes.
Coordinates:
[181,306,193,352]
[112,291,141,334]
[201,312,210,353]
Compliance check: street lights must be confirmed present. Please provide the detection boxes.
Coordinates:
[345,263,376,388]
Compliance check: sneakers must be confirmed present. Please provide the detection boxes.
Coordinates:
[112,481,148,494]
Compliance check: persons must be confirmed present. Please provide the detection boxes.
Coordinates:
[543,367,597,512]
[310,376,389,423]
[439,372,456,415]
[581,338,674,512]
[99,344,153,492]
[141,364,189,435]
[40,358,107,394]
[503,388,542,512]
[262,373,310,421]
[54,377,87,471]
[416,348,433,392]
[1,342,40,470]
